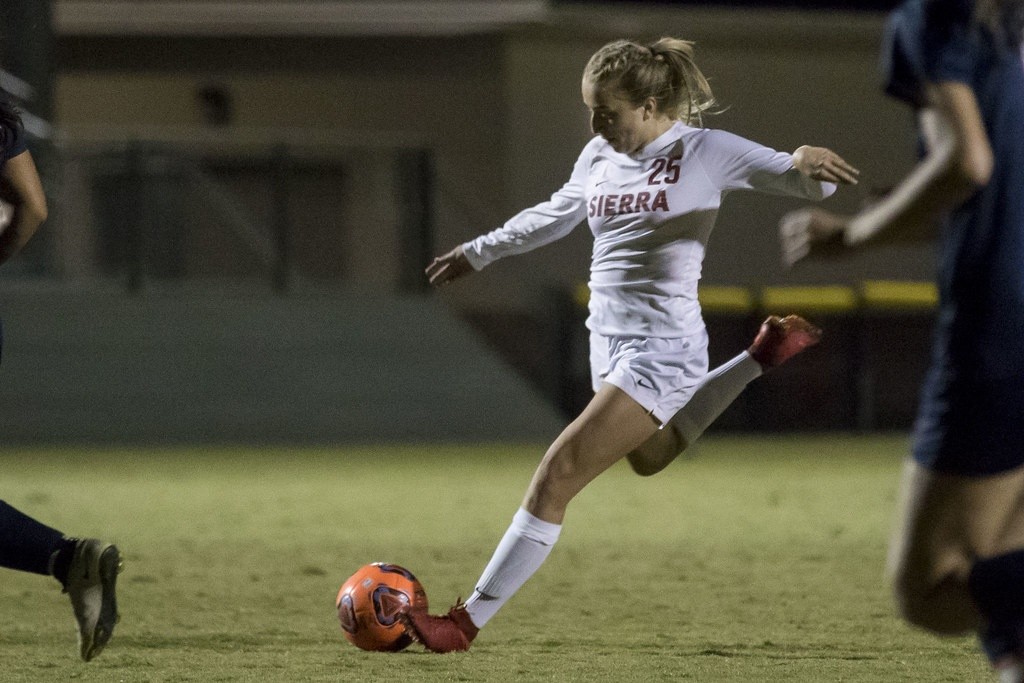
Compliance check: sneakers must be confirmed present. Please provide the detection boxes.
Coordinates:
[401,596,481,653]
[65,536,124,663]
[756,315,822,362]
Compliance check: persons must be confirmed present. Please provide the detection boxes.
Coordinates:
[0,92,125,662]
[778,0,1024,682]
[396,35,863,655]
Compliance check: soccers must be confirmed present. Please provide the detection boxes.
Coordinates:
[333,561,431,654]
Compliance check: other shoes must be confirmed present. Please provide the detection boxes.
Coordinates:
[968,549,1024,683]
